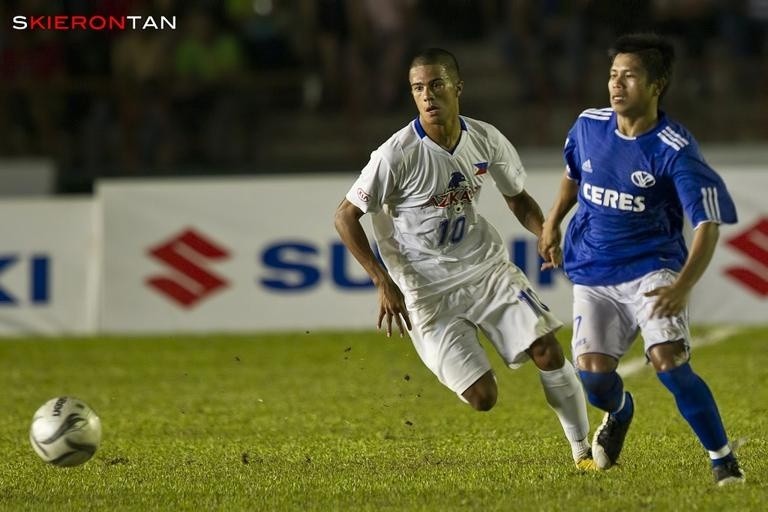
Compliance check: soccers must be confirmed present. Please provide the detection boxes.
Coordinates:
[29,394,101,468]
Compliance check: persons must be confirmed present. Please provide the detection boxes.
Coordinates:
[540,34,748,489]
[1,0,767,200]
[332,45,601,476]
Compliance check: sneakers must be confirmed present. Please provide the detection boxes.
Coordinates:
[713,460,745,487]
[576,411,628,472]
